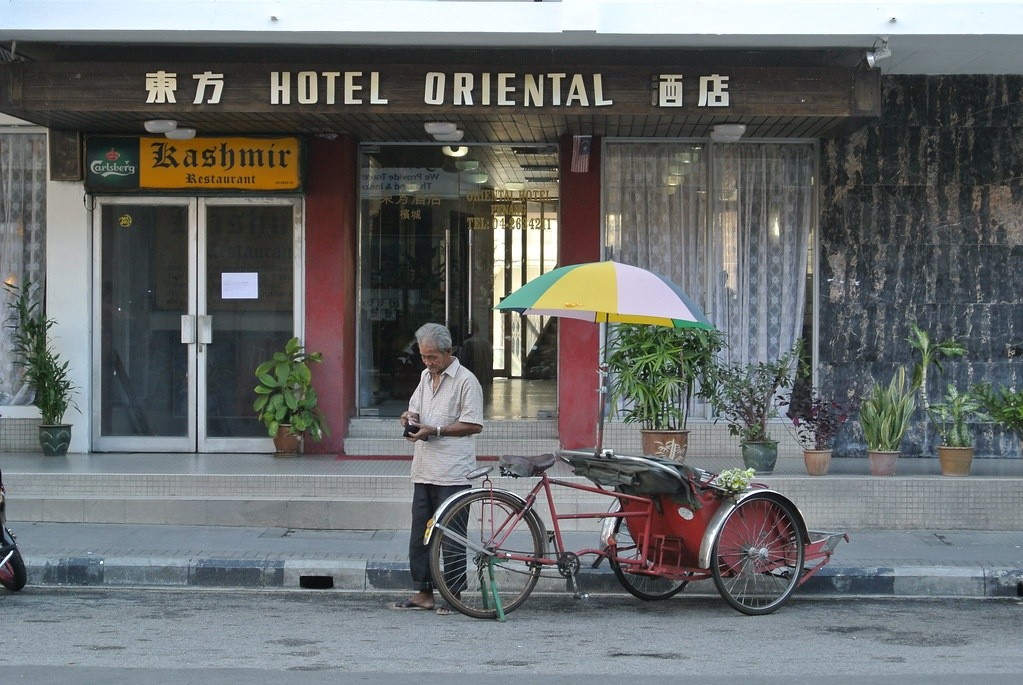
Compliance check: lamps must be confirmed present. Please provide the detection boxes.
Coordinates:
[425,122,456,136]
[165,129,198,140]
[441,146,469,158]
[144,120,180,133]
[454,161,479,170]
[505,182,525,190]
[658,152,698,196]
[467,174,489,184]
[433,130,465,142]
[709,123,746,143]
[865,38,892,67]
[402,183,420,193]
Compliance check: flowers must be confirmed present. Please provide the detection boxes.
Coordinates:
[715,465,757,496]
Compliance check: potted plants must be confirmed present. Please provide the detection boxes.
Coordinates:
[1,279,82,455]
[595,300,730,466]
[902,321,987,477]
[773,378,847,475]
[252,335,330,452]
[858,364,923,477]
[688,336,812,474]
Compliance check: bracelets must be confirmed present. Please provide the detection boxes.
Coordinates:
[436,427,441,440]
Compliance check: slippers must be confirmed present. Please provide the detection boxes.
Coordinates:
[389,599,426,610]
[436,604,460,614]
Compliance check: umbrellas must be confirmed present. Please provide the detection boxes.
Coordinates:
[488,245,717,451]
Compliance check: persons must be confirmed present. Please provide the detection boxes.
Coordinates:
[460,320,492,417]
[386,322,484,615]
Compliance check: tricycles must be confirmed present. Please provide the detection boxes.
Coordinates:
[421,451,849,624]
